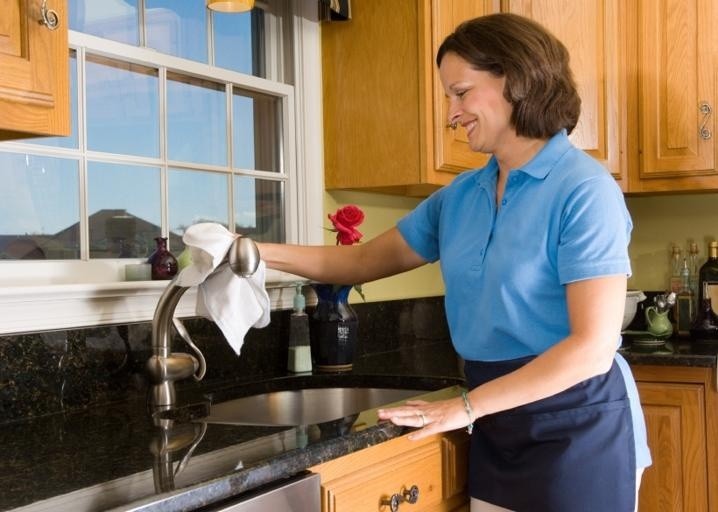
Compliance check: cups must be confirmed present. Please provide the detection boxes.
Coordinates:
[124,263,152,281]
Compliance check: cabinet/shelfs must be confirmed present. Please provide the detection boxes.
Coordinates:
[319,0,502,197]
[0,0,70,141]
[625,366,718,512]
[304,428,475,512]
[623,0,718,195]
[503,0,623,195]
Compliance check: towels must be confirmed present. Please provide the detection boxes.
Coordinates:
[174,222,272,358]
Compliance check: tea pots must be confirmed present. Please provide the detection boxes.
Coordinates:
[644,306,674,340]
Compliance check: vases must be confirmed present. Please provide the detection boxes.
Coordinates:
[307,282,359,376]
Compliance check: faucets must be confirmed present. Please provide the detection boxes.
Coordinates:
[147,416,209,495]
[144,237,261,414]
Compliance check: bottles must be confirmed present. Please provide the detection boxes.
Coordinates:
[697,242,717,315]
[668,242,701,338]
[687,281,717,355]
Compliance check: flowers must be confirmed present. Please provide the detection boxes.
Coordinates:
[322,204,366,303]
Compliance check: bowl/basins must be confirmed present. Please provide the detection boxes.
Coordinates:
[620,289,640,332]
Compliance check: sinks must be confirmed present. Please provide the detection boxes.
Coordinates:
[187,372,467,428]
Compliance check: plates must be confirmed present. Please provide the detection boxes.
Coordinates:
[630,339,664,348]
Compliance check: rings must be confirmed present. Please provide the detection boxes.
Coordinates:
[418,414,425,427]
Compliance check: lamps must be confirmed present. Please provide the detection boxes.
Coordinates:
[206,0,255,13]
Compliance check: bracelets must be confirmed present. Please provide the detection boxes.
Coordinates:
[462,391,474,435]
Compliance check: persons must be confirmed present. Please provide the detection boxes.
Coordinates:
[237,12,654,512]
